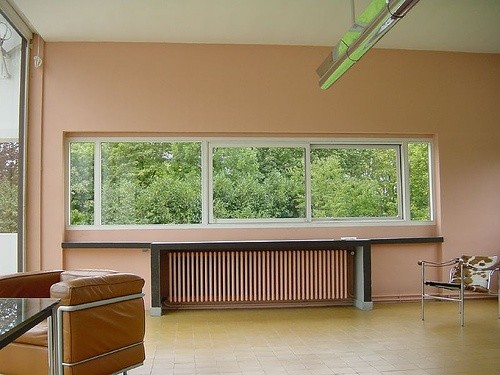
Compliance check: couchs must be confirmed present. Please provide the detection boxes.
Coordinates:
[0,269,146,375]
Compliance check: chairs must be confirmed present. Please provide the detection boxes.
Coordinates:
[417,255,500,327]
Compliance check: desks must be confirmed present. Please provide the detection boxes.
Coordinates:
[0,296,63,375]
[150,238,374,316]
